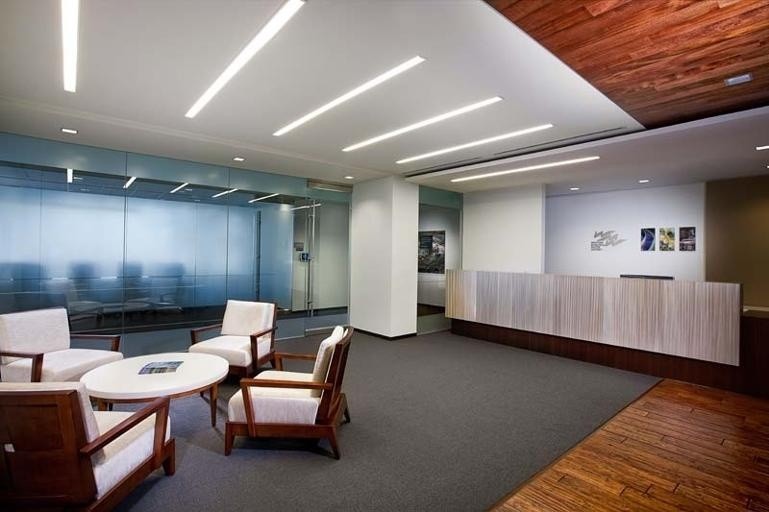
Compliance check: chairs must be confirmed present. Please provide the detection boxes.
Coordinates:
[225,325,354,460]
[0,382,176,511]
[0,306,122,382]
[187,299,280,384]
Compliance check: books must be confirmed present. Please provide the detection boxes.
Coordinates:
[139,361,184,373]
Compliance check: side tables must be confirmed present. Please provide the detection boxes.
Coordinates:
[79,351,229,427]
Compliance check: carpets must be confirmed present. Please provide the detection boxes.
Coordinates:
[72,312,665,512]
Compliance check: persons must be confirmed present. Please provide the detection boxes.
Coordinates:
[663,232,673,248]
